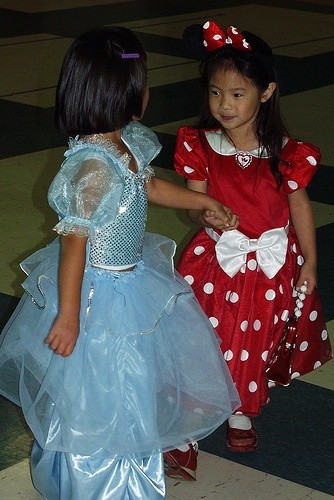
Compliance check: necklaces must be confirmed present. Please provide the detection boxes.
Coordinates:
[222,130,265,170]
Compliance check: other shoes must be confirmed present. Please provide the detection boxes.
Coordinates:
[162,442,199,481]
[224,418,258,452]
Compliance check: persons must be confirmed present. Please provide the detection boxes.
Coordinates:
[0,27,248,500]
[161,18,334,481]
